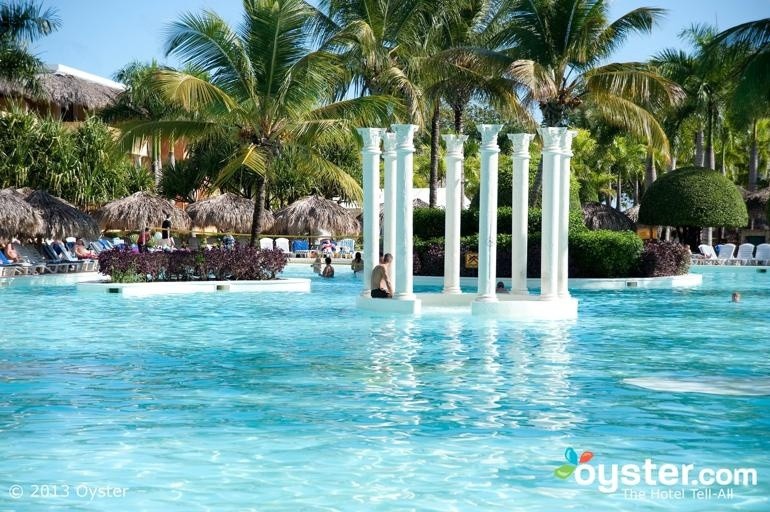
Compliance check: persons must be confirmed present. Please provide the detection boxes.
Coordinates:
[496,281,508,293]
[309,239,336,279]
[351,252,364,274]
[137,227,155,253]
[702,252,712,259]
[4,238,22,262]
[732,292,741,302]
[370,253,393,298]
[75,237,98,260]
[161,213,171,252]
[685,244,693,255]
[379,248,385,263]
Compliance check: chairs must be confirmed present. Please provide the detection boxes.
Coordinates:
[1,237,122,276]
[698,242,769,266]
[259,236,356,259]
